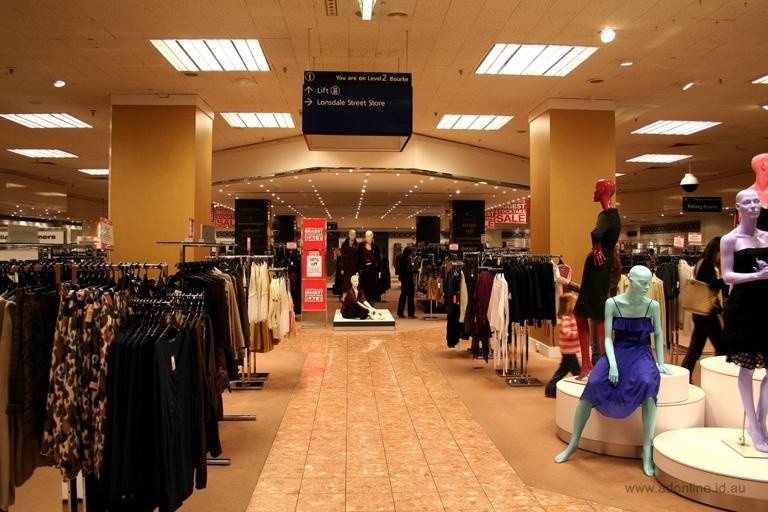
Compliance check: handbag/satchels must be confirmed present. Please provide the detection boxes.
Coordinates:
[680,257,719,317]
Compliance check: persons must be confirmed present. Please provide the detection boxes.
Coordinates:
[553,264,676,480]
[718,188,767,456]
[569,176,622,382]
[339,228,360,304]
[396,244,419,320]
[679,235,726,383]
[556,243,622,365]
[732,153,767,233]
[358,230,382,310]
[542,293,583,399]
[339,274,386,321]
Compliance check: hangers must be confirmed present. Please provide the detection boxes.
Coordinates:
[412,242,706,280]
[0,243,291,350]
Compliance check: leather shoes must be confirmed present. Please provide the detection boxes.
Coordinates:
[396,312,406,318]
[408,315,418,318]
